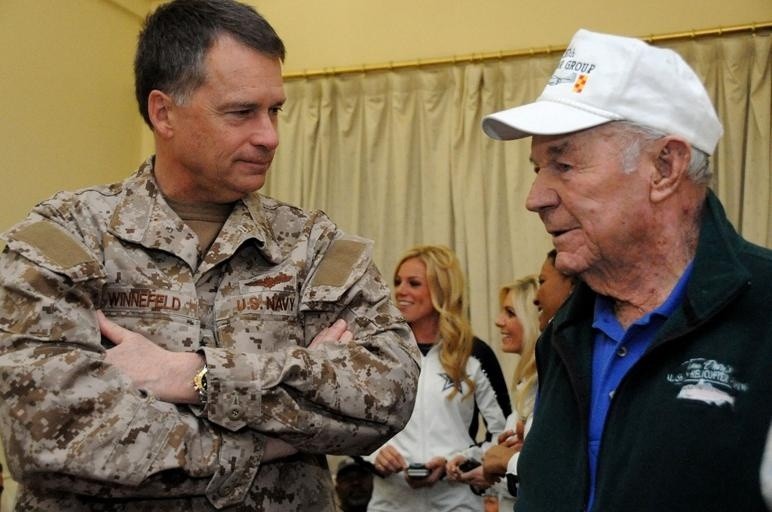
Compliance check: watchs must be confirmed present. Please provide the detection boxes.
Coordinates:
[192,362,208,407]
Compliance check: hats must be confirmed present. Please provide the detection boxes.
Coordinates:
[336,456,370,475]
[482,28,724,157]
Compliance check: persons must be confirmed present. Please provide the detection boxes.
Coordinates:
[484,28,771,512]
[333,250,576,512]
[1,2,420,511]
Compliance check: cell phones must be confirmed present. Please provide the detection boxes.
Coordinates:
[460,457,481,472]
[408,462,430,477]
